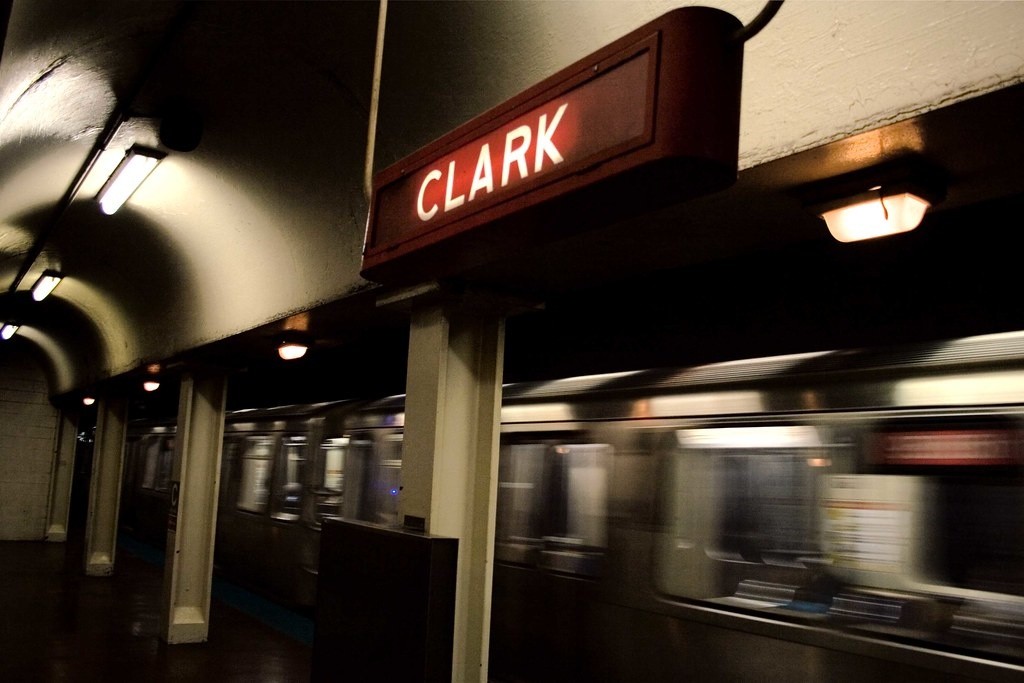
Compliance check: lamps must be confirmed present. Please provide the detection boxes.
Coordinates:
[815,181,947,244]
[94,142,168,215]
[30,268,65,302]
[275,337,314,361]
[0,321,22,340]
